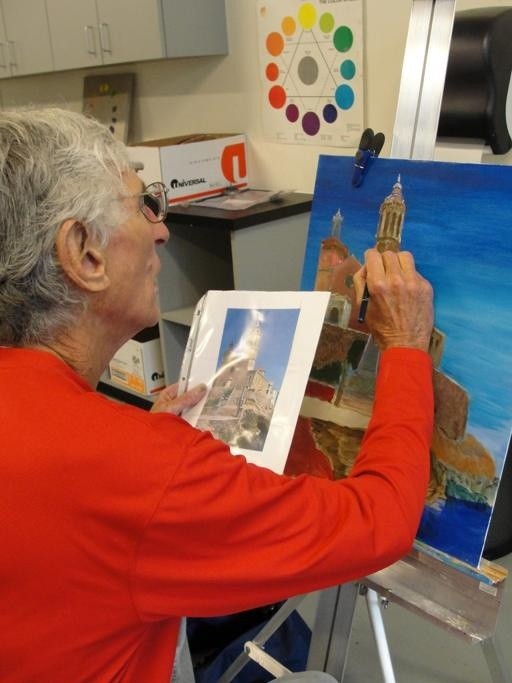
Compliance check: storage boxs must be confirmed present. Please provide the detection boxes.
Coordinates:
[123,131,250,208]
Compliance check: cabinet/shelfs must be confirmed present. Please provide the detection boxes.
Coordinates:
[0,1,228,80]
[99,190,316,417]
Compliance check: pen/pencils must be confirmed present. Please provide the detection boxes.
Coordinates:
[358,239,381,323]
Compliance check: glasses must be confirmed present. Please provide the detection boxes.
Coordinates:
[116,181,169,222]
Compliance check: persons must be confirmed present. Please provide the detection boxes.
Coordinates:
[1,102,437,681]
